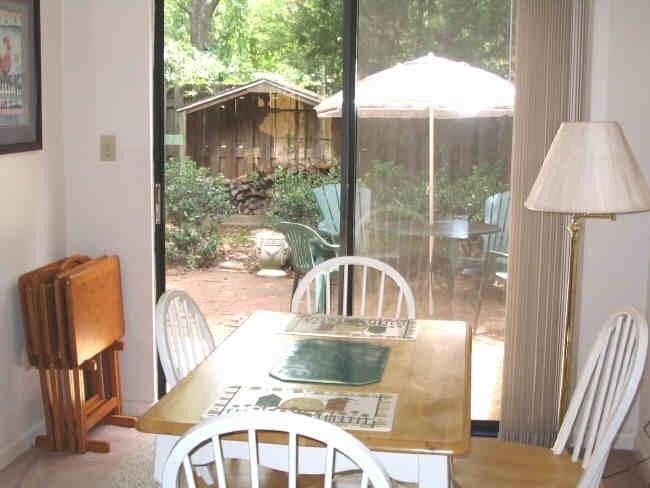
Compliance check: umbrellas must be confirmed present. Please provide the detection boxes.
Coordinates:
[312,50,522,319]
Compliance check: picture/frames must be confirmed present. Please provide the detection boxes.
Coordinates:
[1,0,47,158]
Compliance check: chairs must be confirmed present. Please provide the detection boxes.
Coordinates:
[287,254,416,320]
[161,409,395,487]
[273,179,510,317]
[148,289,233,485]
[447,302,649,486]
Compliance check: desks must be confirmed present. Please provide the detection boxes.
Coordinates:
[130,306,478,487]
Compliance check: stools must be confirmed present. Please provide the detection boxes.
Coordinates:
[16,250,140,459]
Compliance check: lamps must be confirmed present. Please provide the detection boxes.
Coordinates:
[516,118,650,438]
